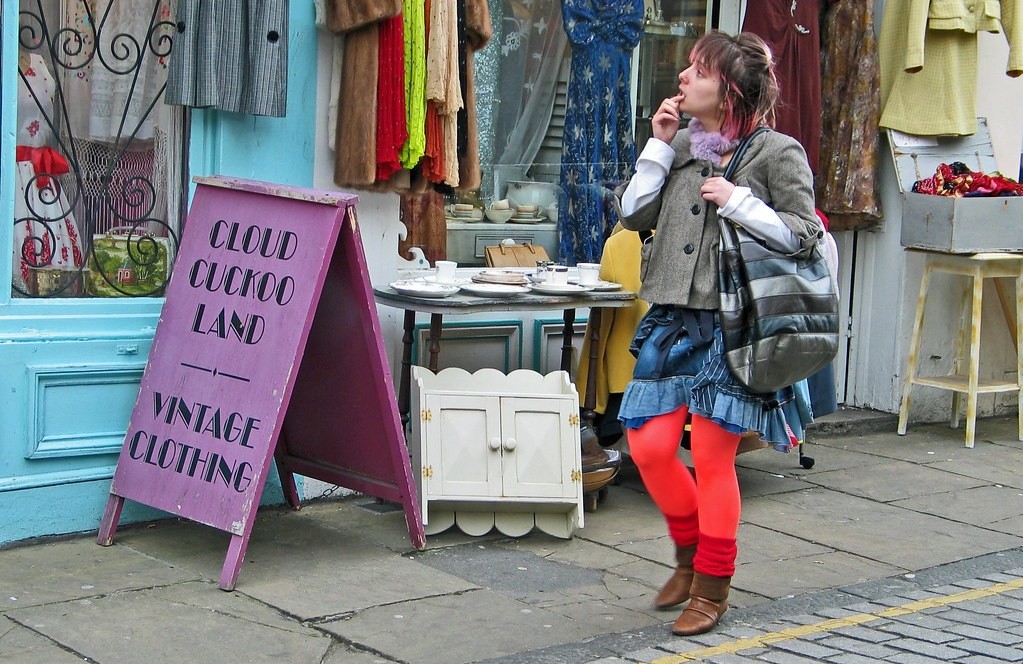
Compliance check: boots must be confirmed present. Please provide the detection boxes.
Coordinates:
[655,544,697,610]
[672,571,731,636]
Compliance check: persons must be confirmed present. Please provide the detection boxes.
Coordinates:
[613,26,823,635]
[15,29,84,284]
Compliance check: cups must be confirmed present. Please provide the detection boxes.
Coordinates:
[434,261,458,282]
[545,265,568,287]
[536,261,554,278]
[490,199,509,210]
[517,206,535,218]
[577,263,602,285]
[454,203,473,218]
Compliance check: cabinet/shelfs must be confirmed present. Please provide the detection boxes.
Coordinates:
[409,365,585,539]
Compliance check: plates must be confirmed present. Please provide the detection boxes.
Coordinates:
[509,217,547,224]
[529,276,545,283]
[531,282,592,295]
[388,280,461,297]
[446,215,481,223]
[471,276,528,285]
[459,283,532,298]
[587,283,623,293]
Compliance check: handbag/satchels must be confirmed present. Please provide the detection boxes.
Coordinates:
[715,126,840,393]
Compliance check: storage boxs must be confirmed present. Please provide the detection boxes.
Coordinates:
[886,116,1023,254]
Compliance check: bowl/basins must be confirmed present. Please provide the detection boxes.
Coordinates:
[485,208,514,224]
[480,269,525,283]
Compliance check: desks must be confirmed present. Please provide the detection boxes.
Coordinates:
[372,286,642,506]
[898,253,1023,449]
